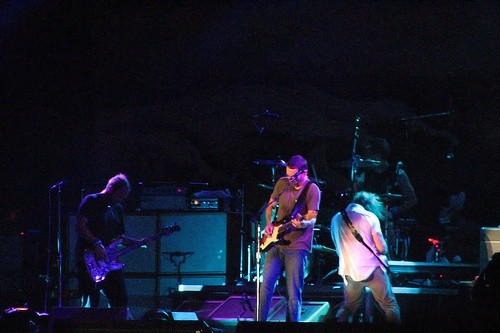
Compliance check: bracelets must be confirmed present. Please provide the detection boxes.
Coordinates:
[91,239,102,247]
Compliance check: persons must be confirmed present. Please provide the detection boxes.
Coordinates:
[331,191,400,323]
[354,145,418,217]
[254,155,321,322]
[75,173,152,307]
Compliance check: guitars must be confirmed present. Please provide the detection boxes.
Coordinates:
[260,216,305,253]
[82,224,181,282]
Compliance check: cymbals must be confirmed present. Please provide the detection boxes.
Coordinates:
[252,159,286,168]
[308,177,328,185]
[334,158,388,168]
[379,193,403,198]
[258,184,274,190]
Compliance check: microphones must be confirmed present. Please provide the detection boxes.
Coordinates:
[254,113,280,120]
[396,162,404,176]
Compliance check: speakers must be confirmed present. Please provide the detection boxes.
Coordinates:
[462,227,500,333]
[51,212,330,333]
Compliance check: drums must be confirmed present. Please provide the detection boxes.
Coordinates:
[381,222,393,259]
[397,218,416,238]
[336,192,355,212]
